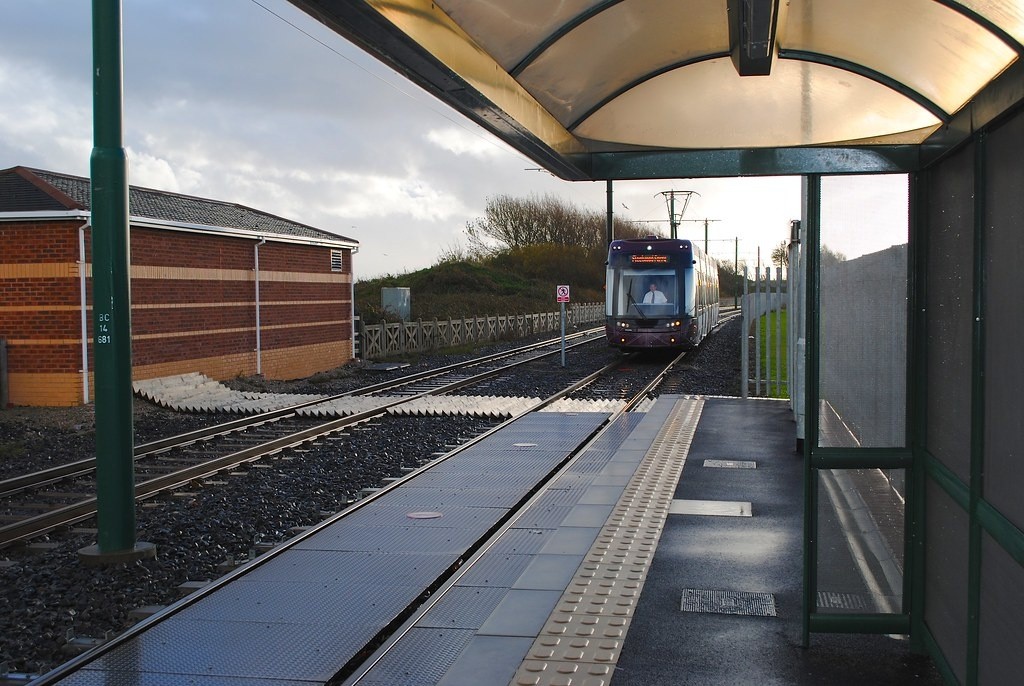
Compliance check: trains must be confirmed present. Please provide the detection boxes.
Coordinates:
[605,189,719,348]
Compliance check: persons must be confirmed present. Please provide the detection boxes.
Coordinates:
[643,283,668,304]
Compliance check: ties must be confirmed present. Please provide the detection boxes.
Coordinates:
[652,292,654,304]
